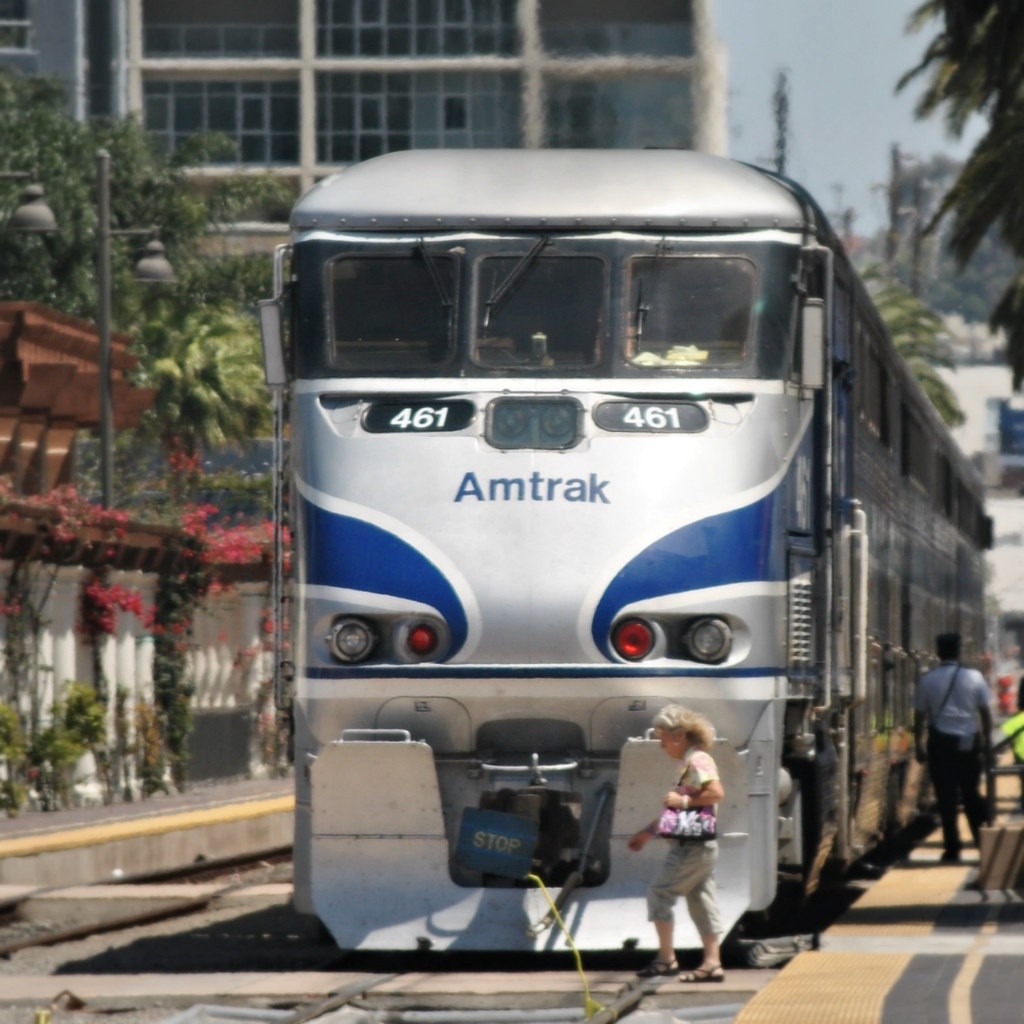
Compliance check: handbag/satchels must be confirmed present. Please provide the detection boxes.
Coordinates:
[658,762,713,839]
[927,724,938,778]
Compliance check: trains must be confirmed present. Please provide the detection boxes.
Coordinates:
[259,141,996,973]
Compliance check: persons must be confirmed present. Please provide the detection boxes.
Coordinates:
[914,631,997,864]
[228,630,300,779]
[628,704,725,983]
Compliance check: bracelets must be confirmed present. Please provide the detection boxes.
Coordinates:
[681,794,690,810]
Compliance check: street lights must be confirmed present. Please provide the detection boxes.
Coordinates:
[93,143,182,518]
[1,168,58,241]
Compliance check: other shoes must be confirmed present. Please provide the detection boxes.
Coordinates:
[942,843,962,861]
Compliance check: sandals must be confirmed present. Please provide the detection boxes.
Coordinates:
[680,965,724,983]
[636,957,680,977]
